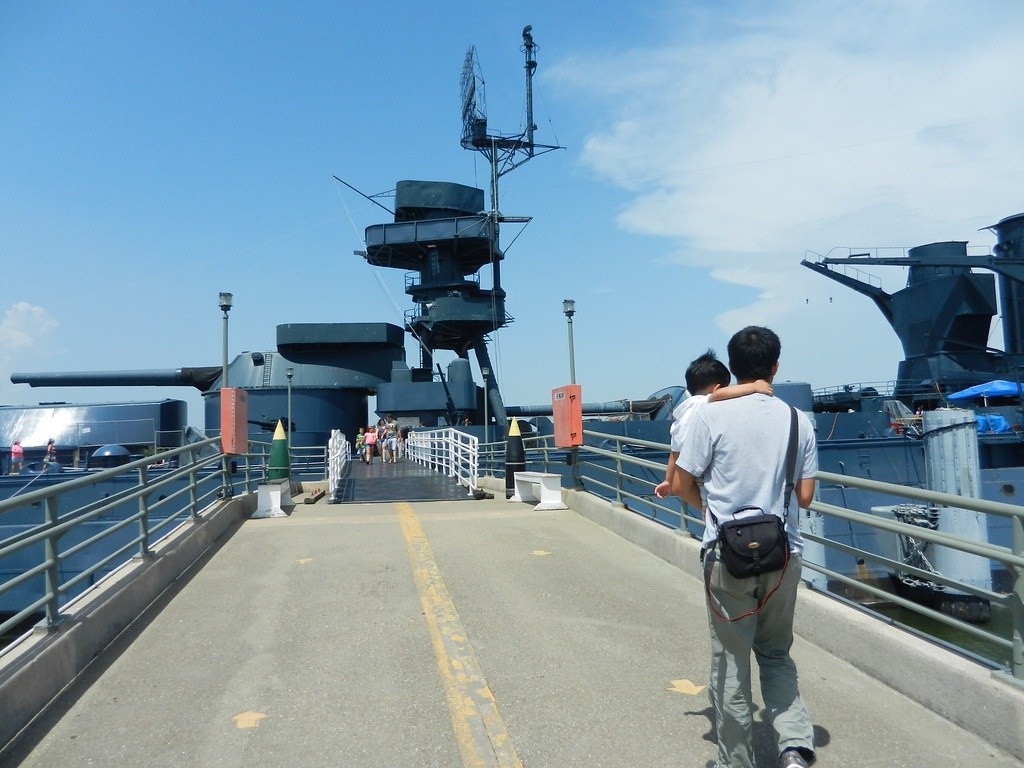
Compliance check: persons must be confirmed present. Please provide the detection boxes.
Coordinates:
[356,414,410,465]
[652,350,774,522]
[11,440,24,473]
[42,438,56,471]
[916,406,924,427]
[464,418,472,427]
[670,324,816,768]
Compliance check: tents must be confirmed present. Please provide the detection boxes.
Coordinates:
[945,379,1024,407]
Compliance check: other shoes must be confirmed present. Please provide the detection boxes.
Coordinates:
[388,459,397,464]
[367,461,369,465]
[360,457,363,463]
[380,459,386,463]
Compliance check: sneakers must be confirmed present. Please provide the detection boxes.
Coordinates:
[782,750,808,768]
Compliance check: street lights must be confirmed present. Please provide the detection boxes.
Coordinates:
[285,367,294,475]
[563,299,587,491]
[481,364,492,477]
[217,290,234,501]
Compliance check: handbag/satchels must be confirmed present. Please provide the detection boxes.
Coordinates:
[717,504,790,579]
